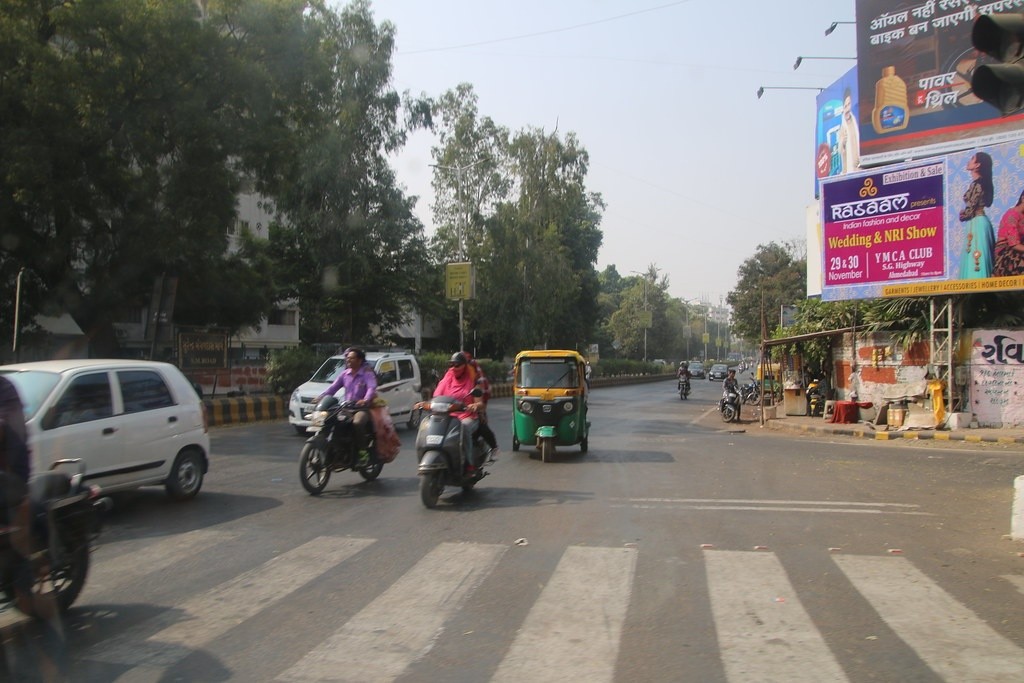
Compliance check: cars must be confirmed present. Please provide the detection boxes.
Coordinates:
[288,348,423,438]
[653,358,722,368]
[0,358,213,505]
[708,363,731,382]
[687,362,707,379]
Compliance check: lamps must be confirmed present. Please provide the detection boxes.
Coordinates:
[793,55,857,71]
[824,21,857,37]
[756,86,825,99]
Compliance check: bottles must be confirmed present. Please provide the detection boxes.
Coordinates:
[872,67,909,134]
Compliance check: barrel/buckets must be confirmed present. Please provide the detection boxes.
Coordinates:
[762,406,776,420]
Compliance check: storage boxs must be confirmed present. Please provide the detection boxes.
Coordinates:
[887,409,906,426]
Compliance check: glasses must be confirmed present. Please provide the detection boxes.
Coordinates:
[449,363,462,368]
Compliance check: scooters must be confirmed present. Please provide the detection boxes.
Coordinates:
[677,370,693,400]
[412,381,497,509]
[808,386,826,417]
[739,361,753,374]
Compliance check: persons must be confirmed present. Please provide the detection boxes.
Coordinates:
[563,375,588,415]
[0,376,30,508]
[313,348,378,469]
[991,190,1024,278]
[677,364,692,393]
[959,152,995,279]
[723,369,741,419]
[838,86,860,173]
[414,352,499,479]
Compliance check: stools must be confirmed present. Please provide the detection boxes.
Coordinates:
[822,400,836,420]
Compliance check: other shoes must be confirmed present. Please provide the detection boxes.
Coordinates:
[465,470,475,478]
[354,449,371,468]
[488,447,500,460]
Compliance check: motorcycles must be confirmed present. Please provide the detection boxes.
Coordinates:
[0,455,109,619]
[717,379,742,423]
[755,362,783,397]
[298,394,386,496]
[511,349,593,463]
[739,372,761,406]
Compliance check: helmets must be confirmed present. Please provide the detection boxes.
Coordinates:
[448,352,466,363]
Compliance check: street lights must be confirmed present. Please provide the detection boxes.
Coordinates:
[680,297,698,362]
[631,268,662,362]
[428,157,490,350]
